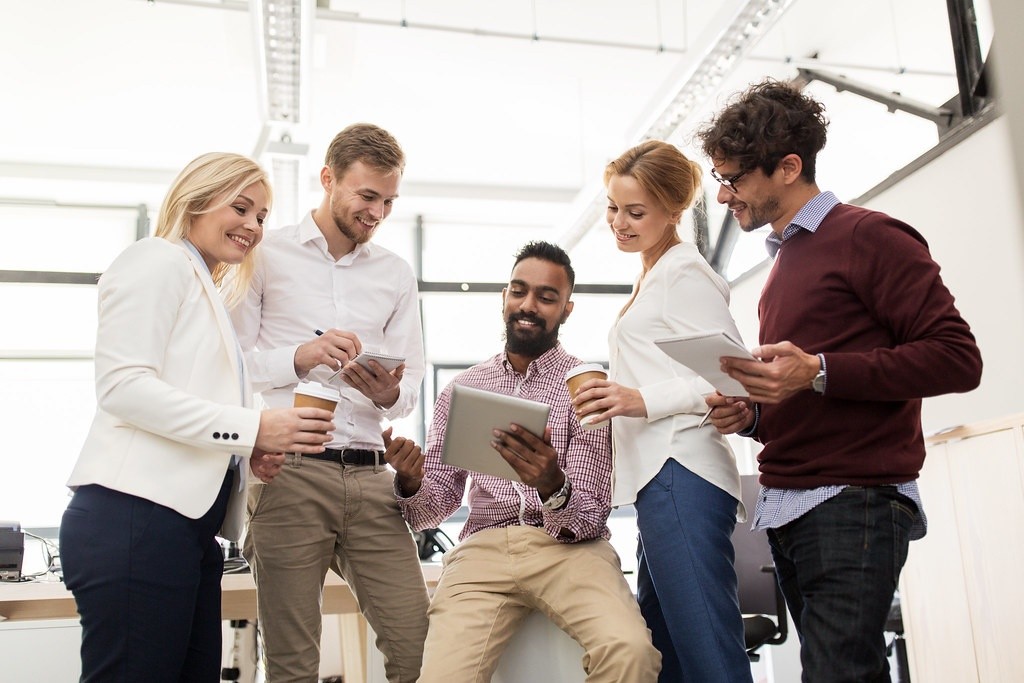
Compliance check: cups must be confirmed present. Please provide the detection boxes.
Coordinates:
[564,363,610,429]
[293,381,340,449]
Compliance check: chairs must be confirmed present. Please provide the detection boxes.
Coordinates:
[729,474,788,662]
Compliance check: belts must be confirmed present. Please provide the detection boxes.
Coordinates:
[288,448,386,467]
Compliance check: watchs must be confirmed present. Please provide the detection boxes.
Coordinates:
[813,371,825,393]
[537,468,570,509]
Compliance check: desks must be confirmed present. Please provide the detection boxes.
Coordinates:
[0,564,634,683]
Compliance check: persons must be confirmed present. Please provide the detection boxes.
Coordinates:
[59,152,335,683]
[219,126,425,683]
[570,143,750,683]
[705,84,982,683]
[381,241,662,683]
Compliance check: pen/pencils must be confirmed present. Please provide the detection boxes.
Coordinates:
[698,407,716,428]
[313,328,358,356]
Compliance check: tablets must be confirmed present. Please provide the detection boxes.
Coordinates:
[441,384,550,483]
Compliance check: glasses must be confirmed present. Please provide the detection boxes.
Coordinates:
[709,168,746,196]
